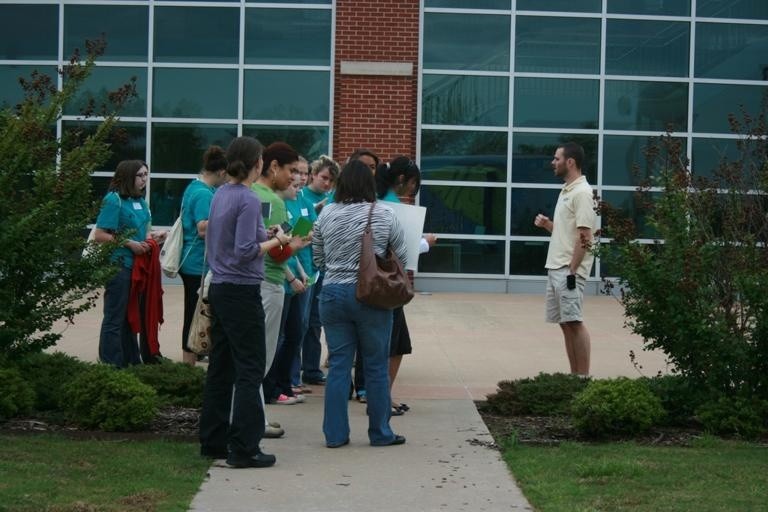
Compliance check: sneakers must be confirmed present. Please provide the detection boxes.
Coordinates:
[371,434,405,446]
[276,376,410,416]
[201,421,284,467]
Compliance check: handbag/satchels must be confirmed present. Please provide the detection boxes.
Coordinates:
[82,224,103,264]
[356,226,414,310]
[186,296,212,356]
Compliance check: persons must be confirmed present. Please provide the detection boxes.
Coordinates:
[178,132,438,446]
[198,134,291,466]
[92,162,168,367]
[534,142,597,376]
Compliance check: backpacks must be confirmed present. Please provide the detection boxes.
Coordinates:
[158,189,213,278]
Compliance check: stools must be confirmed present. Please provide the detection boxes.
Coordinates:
[429,244,462,273]
